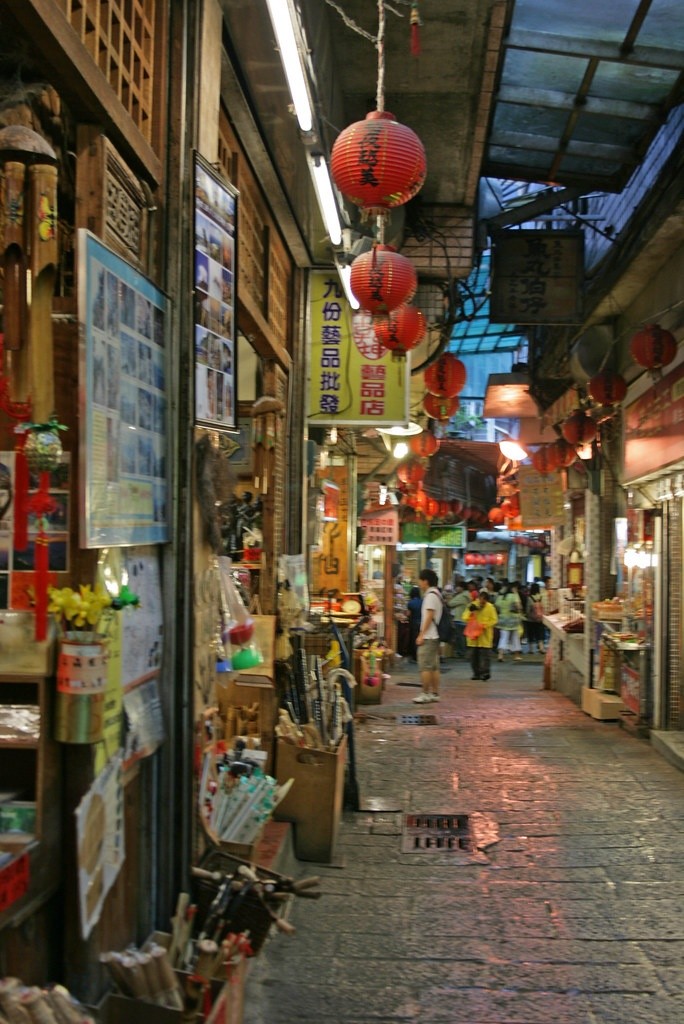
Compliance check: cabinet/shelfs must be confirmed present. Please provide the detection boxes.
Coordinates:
[0,676,62,985]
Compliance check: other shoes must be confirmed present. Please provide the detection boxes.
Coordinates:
[537,649,546,654]
[512,657,523,661]
[528,649,534,654]
[498,654,504,662]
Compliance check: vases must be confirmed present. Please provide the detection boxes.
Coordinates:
[51,632,107,746]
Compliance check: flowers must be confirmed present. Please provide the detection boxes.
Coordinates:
[43,586,113,627]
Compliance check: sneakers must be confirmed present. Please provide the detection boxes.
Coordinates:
[411,693,440,703]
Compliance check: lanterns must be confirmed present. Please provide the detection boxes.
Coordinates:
[422,351,466,427]
[396,429,521,524]
[351,244,418,325]
[374,303,427,388]
[532,410,597,481]
[464,552,506,567]
[629,322,677,400]
[514,537,543,550]
[330,111,427,269]
[590,368,628,408]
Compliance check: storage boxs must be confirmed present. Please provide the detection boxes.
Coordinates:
[97,928,246,1024]
[276,731,350,862]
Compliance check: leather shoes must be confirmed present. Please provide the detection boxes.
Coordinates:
[471,674,490,680]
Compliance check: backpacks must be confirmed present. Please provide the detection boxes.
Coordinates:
[530,599,544,622]
[436,605,457,644]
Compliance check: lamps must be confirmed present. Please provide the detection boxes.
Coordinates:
[335,252,361,315]
[266,1,322,144]
[499,435,534,463]
[308,151,344,252]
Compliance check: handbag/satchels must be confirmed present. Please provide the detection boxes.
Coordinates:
[463,613,485,640]
[499,614,520,628]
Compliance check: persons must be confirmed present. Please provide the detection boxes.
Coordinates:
[406,575,554,662]
[462,591,498,681]
[412,569,445,703]
